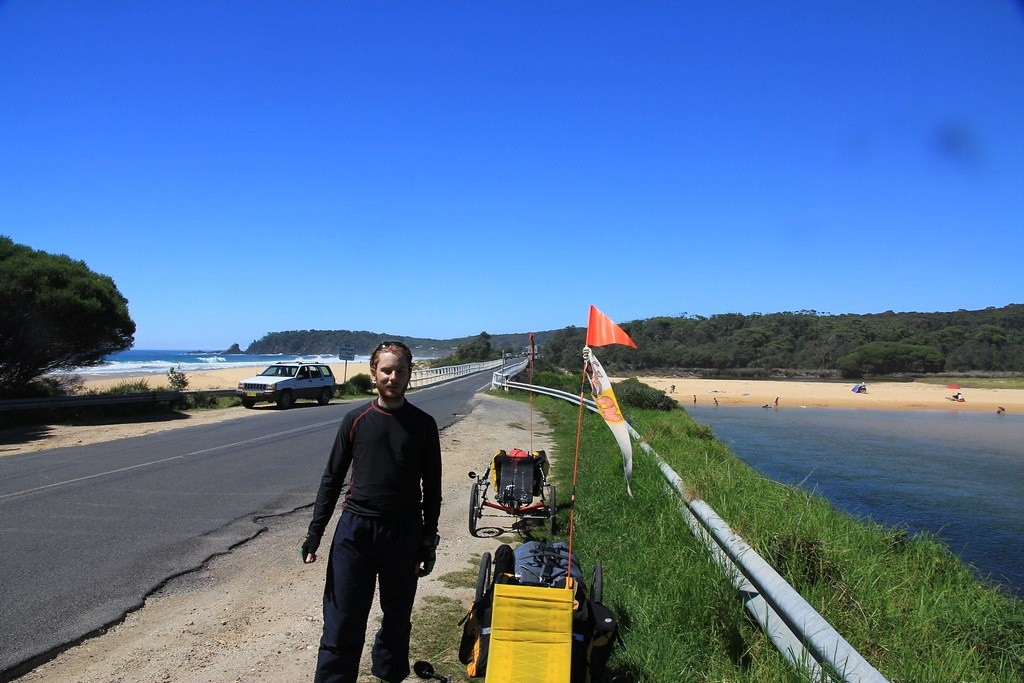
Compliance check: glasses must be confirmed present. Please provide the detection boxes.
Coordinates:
[372,341,412,363]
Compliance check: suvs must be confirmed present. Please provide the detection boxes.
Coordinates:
[236,360,337,408]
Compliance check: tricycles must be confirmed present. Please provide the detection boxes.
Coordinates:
[468,450,558,535]
[471,532,602,683]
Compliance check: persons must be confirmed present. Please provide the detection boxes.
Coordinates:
[693,395,696,403]
[713,398,718,406]
[301,342,442,683]
[958,393,966,402]
[997,407,1005,415]
[775,396,779,406]
[671,385,675,392]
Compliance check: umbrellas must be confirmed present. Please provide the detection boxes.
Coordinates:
[947,384,960,397]
